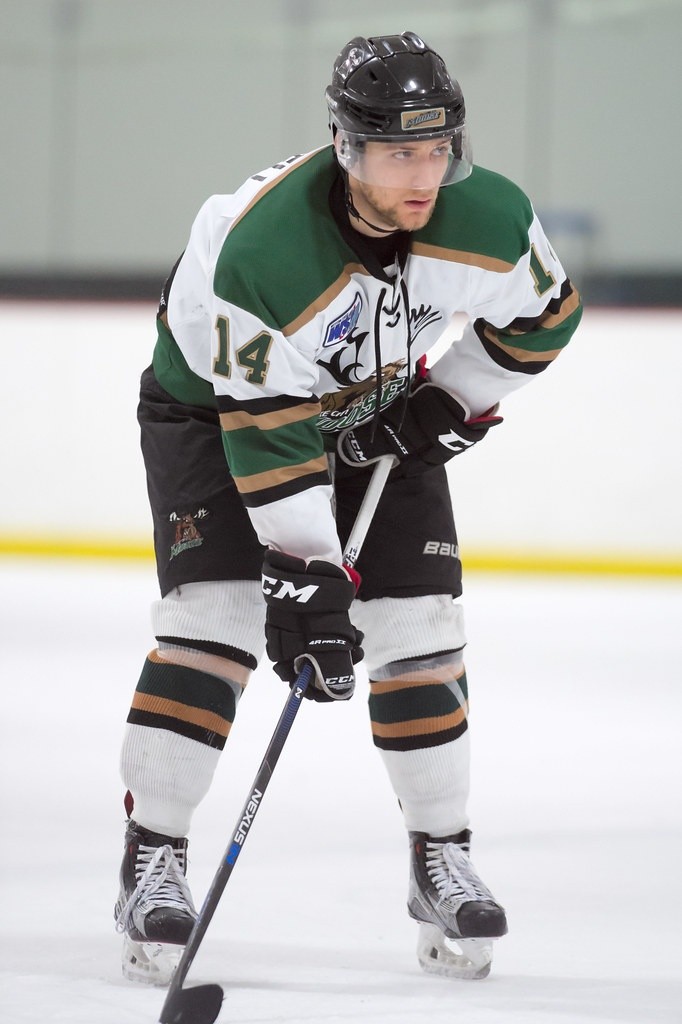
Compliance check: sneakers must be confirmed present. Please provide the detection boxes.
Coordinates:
[114,791,200,987]
[408,830,507,981]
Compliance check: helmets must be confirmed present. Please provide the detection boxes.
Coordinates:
[325,31,465,170]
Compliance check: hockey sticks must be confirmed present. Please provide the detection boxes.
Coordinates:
[159,306,475,1024]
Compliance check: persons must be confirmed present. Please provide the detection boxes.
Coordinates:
[116,32,584,988]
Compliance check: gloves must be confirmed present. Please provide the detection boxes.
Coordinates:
[338,353,504,491]
[261,548,365,702]
